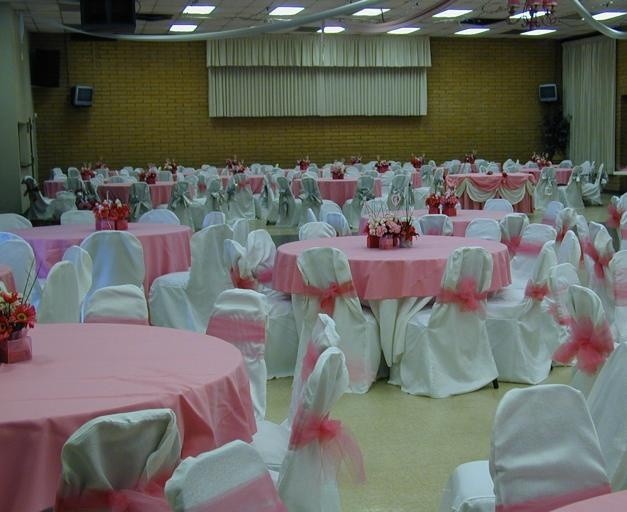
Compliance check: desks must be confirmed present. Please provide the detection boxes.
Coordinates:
[1,322,257,512]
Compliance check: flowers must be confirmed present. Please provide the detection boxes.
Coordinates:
[1,288,37,334]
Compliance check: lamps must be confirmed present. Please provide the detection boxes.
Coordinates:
[506,1,558,28]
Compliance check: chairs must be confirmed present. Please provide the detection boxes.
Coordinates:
[50,406,186,512]
[165,437,287,511]
[276,344,356,512]
[446,343,627,512]
[2,164,626,400]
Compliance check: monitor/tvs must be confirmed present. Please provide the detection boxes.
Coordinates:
[538,83,557,102]
[71,85,93,107]
